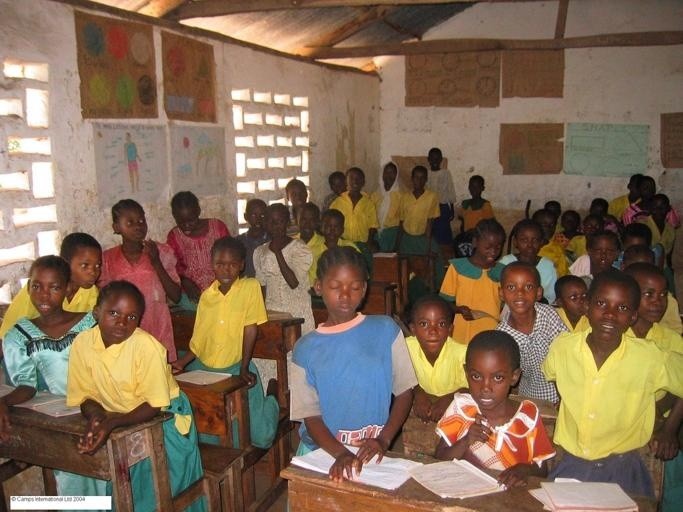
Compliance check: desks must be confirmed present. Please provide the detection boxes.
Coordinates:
[1,248,683,511]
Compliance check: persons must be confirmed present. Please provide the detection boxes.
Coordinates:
[430,329,557,489]
[287,245,418,512]
[0,233,104,359]
[95,199,181,364]
[122,131,143,194]
[538,268,682,500]
[0,255,106,511]
[64,280,210,511]
[598,262,682,511]
